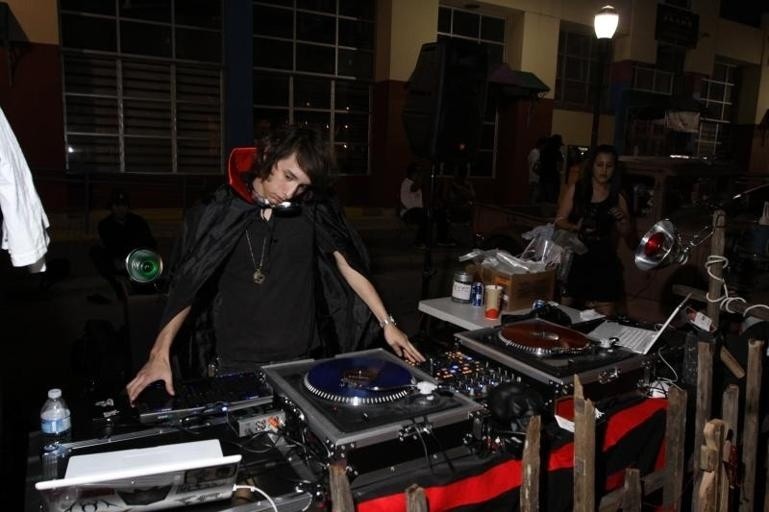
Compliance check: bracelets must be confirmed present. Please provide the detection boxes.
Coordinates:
[378,315,396,330]
[620,222,628,225]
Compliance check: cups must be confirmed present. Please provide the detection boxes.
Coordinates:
[484,284,502,320]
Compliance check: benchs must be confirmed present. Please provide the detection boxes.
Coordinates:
[394,176,478,242]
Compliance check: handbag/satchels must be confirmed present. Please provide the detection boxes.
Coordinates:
[533,235,575,288]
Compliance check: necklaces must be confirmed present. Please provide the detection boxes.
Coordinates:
[246,229,270,285]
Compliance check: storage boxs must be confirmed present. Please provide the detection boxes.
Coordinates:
[475,263,554,313]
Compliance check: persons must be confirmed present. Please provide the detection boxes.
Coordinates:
[96,191,158,257]
[126,121,427,404]
[525,136,550,204]
[399,158,439,250]
[442,163,481,225]
[554,143,631,322]
[531,132,567,203]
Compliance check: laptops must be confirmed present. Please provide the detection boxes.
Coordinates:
[586,290,693,356]
[34,436,244,512]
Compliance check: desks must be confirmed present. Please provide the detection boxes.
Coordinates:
[417,296,606,332]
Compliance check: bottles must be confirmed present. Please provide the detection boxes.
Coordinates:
[40,388,73,480]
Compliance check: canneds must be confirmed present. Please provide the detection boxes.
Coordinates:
[532,300,545,310]
[471,282,484,306]
[450,272,473,304]
